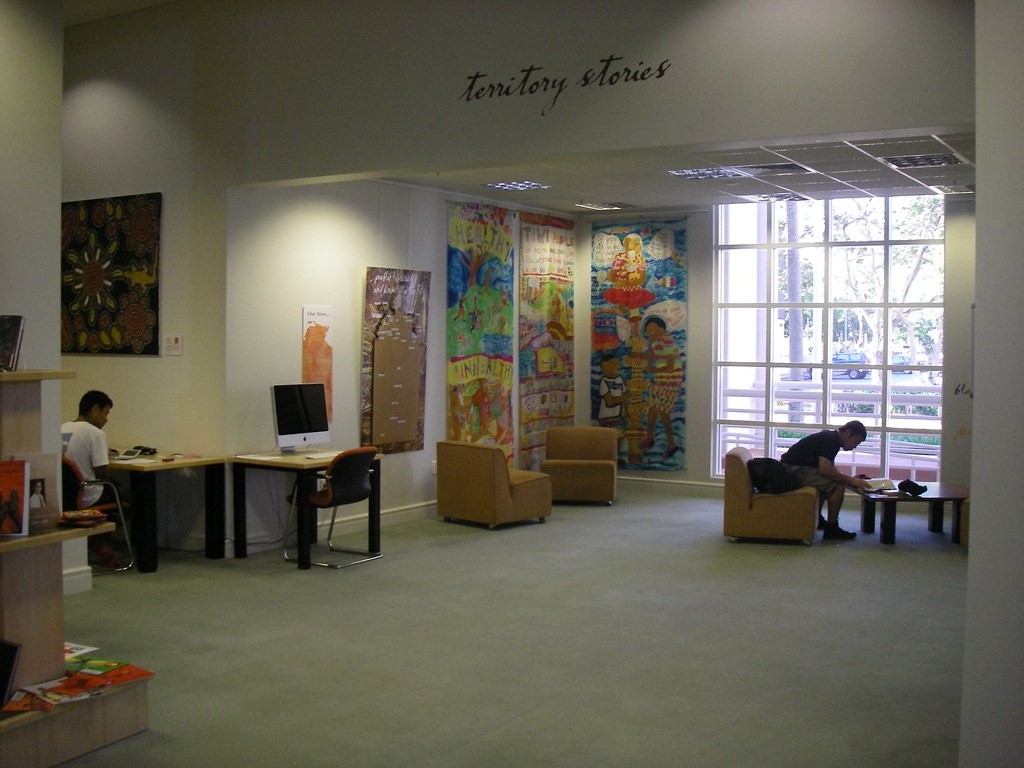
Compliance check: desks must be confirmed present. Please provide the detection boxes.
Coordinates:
[229,448,385,569]
[107,443,225,572]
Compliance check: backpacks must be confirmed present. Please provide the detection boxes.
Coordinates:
[747,458,800,494]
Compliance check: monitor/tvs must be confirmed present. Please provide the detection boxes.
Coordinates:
[271,383,330,453]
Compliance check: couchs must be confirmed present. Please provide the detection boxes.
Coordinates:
[437,440,552,529]
[725,446,817,543]
[541,426,618,504]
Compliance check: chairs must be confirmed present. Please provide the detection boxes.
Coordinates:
[61,455,134,576]
[282,445,383,567]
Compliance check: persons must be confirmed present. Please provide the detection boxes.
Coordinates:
[779,421,873,541]
[30,481,47,508]
[60,388,132,568]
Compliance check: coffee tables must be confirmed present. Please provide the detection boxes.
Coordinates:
[848,481,969,543]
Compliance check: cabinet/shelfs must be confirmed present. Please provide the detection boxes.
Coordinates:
[0,369,157,767]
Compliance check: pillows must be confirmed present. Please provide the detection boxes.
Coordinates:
[747,457,800,493]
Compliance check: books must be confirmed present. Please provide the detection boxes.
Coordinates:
[0,637,157,714]
[0,314,26,372]
[863,479,898,492]
[0,460,31,537]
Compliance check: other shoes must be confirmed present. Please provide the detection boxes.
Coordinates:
[823,521,856,541]
[817,514,828,530]
[87,539,113,559]
[95,554,122,568]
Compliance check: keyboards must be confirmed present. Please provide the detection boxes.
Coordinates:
[305,450,345,459]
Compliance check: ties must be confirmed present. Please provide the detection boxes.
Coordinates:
[39,496,43,507]
